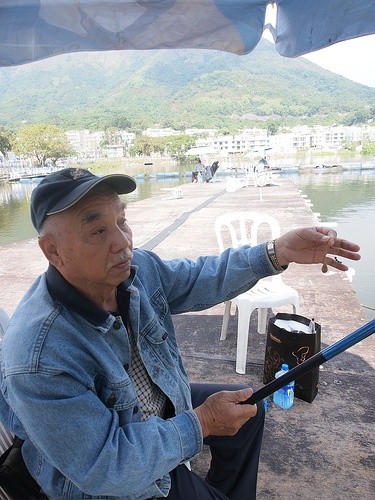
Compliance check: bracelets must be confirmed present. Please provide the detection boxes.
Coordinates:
[267,239,283,270]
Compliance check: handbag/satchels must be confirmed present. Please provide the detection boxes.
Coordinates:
[263,313,321,404]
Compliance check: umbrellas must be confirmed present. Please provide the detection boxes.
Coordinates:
[0,0,375,67]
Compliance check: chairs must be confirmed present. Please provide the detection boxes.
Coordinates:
[220,212,299,374]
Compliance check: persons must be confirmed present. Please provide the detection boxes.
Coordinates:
[0,169,361,500]
[191,159,220,183]
[258,155,267,165]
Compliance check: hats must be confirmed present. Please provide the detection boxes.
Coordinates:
[30,168,136,234]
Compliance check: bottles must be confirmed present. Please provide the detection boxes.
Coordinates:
[274,363,294,411]
